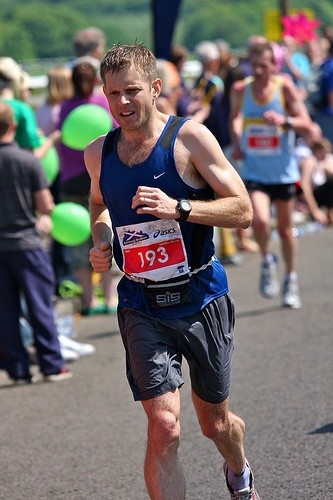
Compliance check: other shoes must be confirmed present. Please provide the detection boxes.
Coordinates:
[57,333,95,363]
[11,367,73,384]
[79,301,117,315]
[280,272,302,308]
[258,254,280,298]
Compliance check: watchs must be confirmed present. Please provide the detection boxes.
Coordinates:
[174,198,192,222]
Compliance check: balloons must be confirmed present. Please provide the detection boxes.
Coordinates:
[33,137,59,186]
[49,202,90,245]
[62,104,110,150]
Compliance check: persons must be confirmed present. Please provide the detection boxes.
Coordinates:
[228,42,310,309]
[82,42,259,500]
[0,28,333,369]
[0,104,73,384]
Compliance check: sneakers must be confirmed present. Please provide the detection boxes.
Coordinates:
[224,456,260,500]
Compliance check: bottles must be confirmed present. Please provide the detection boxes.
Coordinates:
[53,298,76,336]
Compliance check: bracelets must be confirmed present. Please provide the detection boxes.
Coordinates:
[285,116,291,130]
